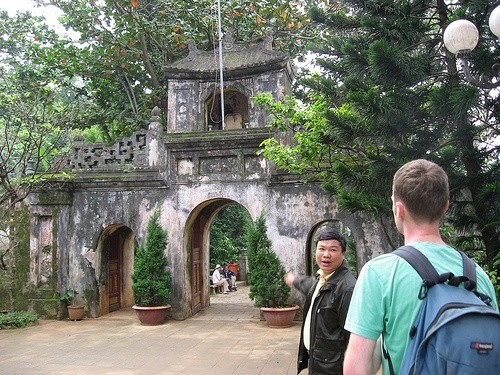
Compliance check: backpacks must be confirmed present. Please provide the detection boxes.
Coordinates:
[380,245,500,375]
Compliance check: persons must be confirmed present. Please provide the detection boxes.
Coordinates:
[343,158,500,375]
[223,264,237,291]
[212,264,232,294]
[228,261,239,276]
[284,228,356,375]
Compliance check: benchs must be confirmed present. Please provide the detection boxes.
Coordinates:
[210,284,229,294]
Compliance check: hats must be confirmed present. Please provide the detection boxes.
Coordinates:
[215,263,222,269]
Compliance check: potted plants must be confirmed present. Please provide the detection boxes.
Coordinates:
[246,205,299,327]
[62,285,84,321]
[131,209,173,325]
